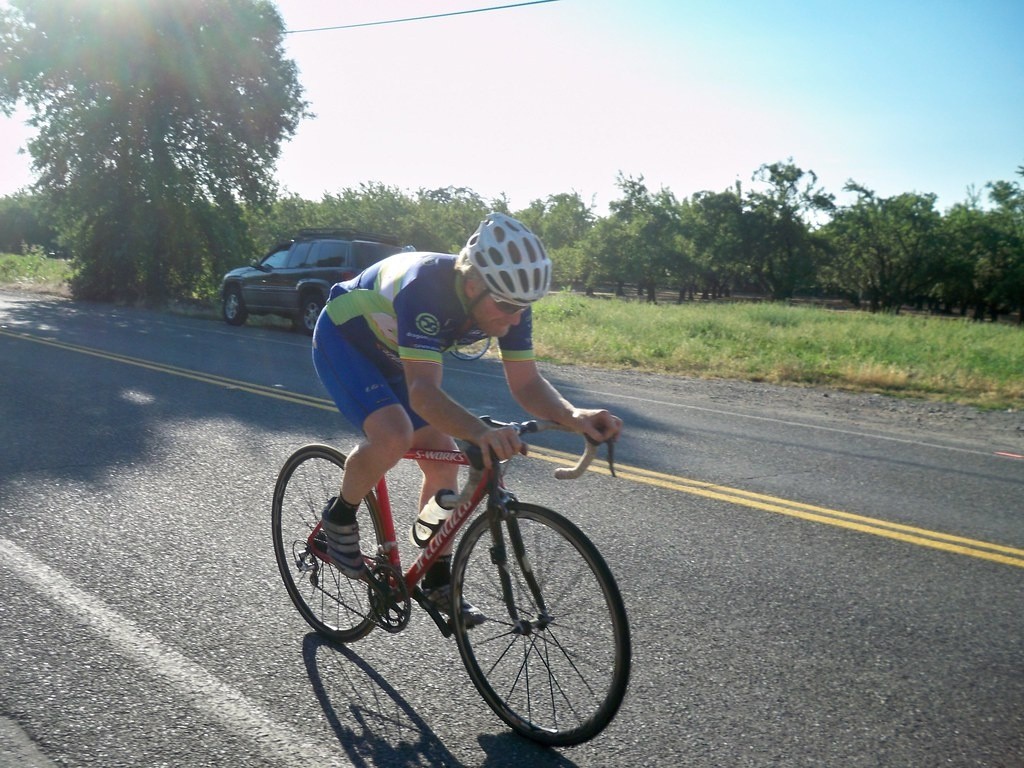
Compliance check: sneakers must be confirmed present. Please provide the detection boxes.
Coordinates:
[321,497,367,579]
[420,575,487,625]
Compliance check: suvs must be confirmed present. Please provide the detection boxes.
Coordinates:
[221,227,414,336]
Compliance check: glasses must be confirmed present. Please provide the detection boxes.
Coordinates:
[494,301,527,315]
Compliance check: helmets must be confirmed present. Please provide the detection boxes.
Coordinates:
[466,211,552,308]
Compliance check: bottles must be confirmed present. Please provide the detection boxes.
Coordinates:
[407,489,457,550]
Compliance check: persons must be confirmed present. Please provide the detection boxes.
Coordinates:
[310,211,623,623]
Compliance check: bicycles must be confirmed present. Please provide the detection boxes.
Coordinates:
[271,415,632,749]
[447,336,491,363]
